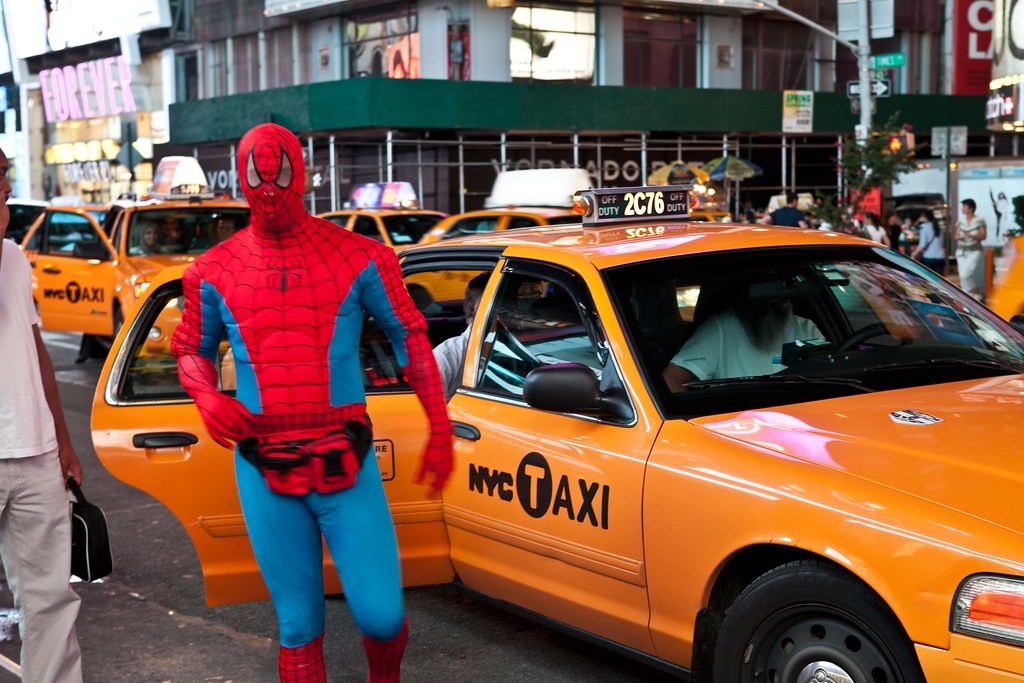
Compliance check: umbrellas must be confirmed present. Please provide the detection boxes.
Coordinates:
[702,156,762,212]
[648,160,709,185]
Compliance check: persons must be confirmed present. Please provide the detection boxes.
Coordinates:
[129,214,234,254]
[663,282,826,393]
[168,123,457,683]
[740,193,861,237]
[990,191,1007,237]
[0,150,114,683]
[432,272,521,402]
[864,197,987,305]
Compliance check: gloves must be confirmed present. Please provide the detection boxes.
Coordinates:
[412,422,458,502]
[197,386,259,450]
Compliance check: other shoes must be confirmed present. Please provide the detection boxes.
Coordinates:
[975,293,984,302]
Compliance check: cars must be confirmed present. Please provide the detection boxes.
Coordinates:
[6,155,603,387]
[90,183,1024,683]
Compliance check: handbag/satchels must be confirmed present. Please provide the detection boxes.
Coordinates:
[237,419,374,496]
[62,476,113,582]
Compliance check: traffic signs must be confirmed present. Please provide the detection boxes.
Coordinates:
[847,79,891,99]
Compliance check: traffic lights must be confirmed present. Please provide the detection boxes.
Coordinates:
[886,135,908,156]
